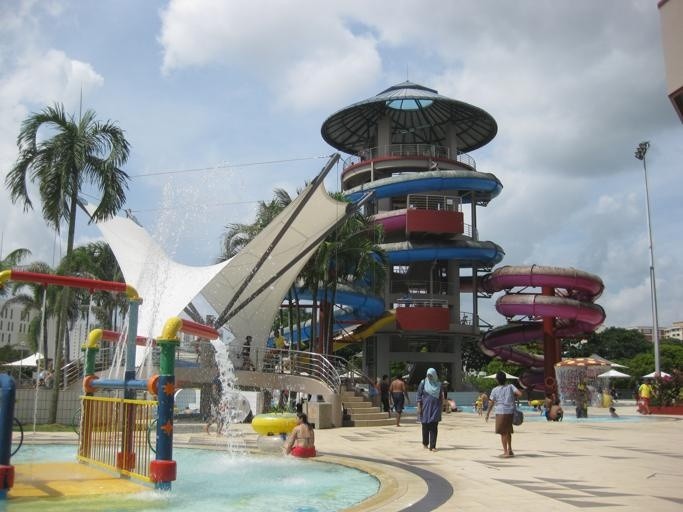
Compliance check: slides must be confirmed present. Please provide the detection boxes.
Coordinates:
[267,173,506,402]
[283,309,397,371]
[364,206,605,399]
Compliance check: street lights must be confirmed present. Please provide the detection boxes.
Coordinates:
[635,141,663,393]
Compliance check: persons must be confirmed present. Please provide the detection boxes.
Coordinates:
[415,367,444,452]
[484,371,522,458]
[194,330,410,435]
[282,413,316,457]
[447,398,457,413]
[400,292,413,307]
[530,392,564,422]
[609,407,618,416]
[637,379,658,415]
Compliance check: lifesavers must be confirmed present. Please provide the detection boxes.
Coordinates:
[250,412,300,434]
[258,436,288,451]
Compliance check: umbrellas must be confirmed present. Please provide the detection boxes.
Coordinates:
[642,370,671,378]
[596,368,632,379]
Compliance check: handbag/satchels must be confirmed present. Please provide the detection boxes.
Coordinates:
[512,392,522,425]
